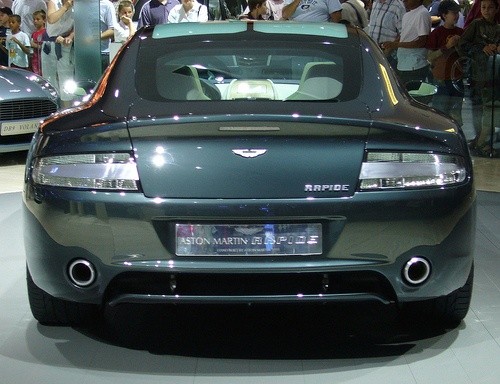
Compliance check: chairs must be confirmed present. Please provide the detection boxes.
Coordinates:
[299,61,343,99]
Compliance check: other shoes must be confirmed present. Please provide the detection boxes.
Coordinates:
[468,136,491,151]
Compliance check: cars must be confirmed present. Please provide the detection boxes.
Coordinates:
[20,21,477,346]
[0,64,65,155]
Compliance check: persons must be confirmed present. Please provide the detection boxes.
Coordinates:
[0,0,135,109]
[339,0,500,159]
[137,0,343,79]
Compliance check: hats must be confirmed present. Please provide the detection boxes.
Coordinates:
[438,0,461,15]
